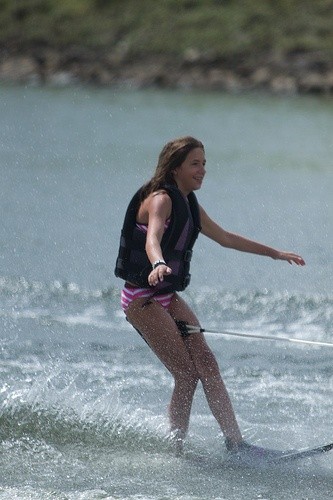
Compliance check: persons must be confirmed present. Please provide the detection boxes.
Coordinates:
[113,135,305,458]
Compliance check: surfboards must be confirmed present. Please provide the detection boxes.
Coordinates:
[265,442,333,468]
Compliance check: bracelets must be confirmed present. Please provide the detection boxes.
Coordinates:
[150,259,166,268]
